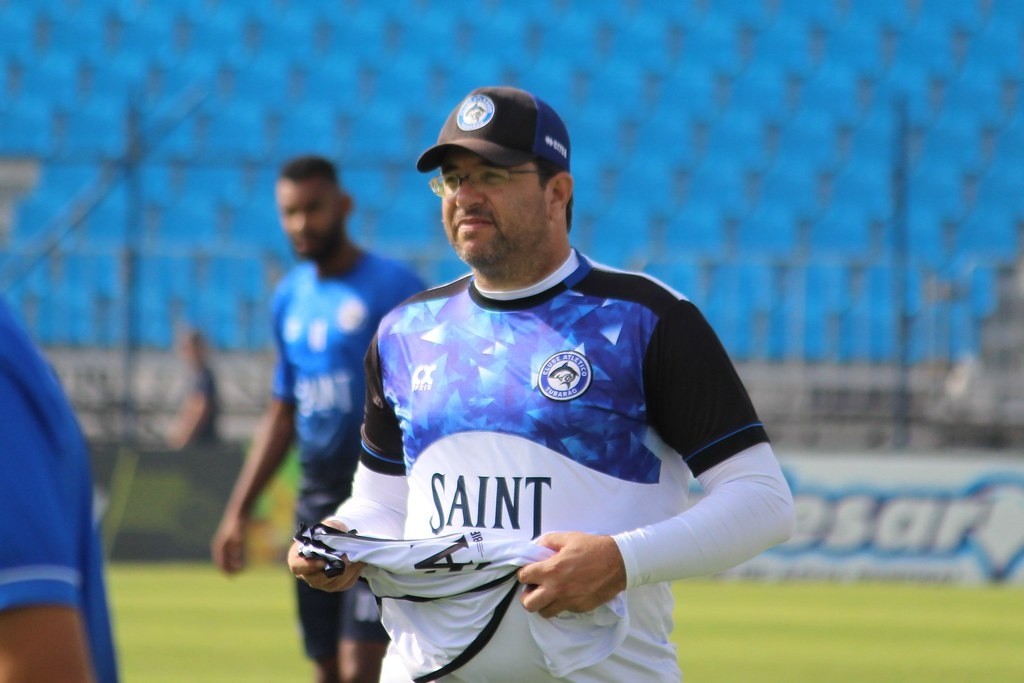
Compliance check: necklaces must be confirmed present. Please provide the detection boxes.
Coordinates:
[211,154,429,683]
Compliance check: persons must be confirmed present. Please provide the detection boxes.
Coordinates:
[0,291,118,683]
[287,88,797,683]
[169,329,231,527]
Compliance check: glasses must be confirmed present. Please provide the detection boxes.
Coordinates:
[429,169,539,198]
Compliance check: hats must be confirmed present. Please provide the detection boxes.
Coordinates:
[416,85,571,173]
